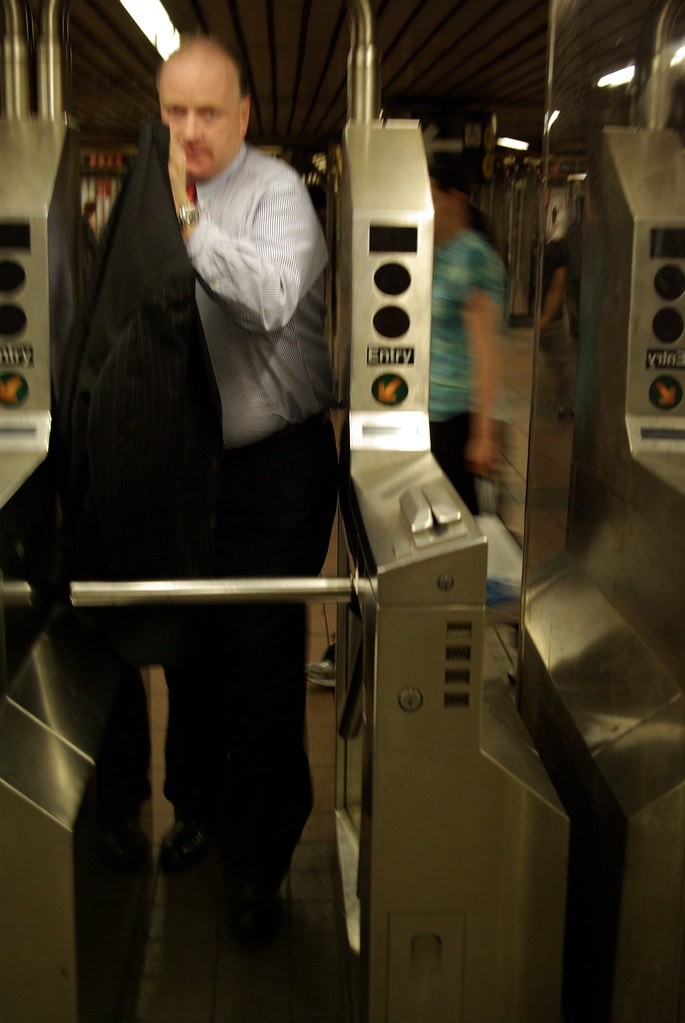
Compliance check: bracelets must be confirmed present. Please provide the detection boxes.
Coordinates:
[178,202,199,229]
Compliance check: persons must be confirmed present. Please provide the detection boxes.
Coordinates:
[152,39,337,955]
[306,156,501,689]
[529,208,577,419]
